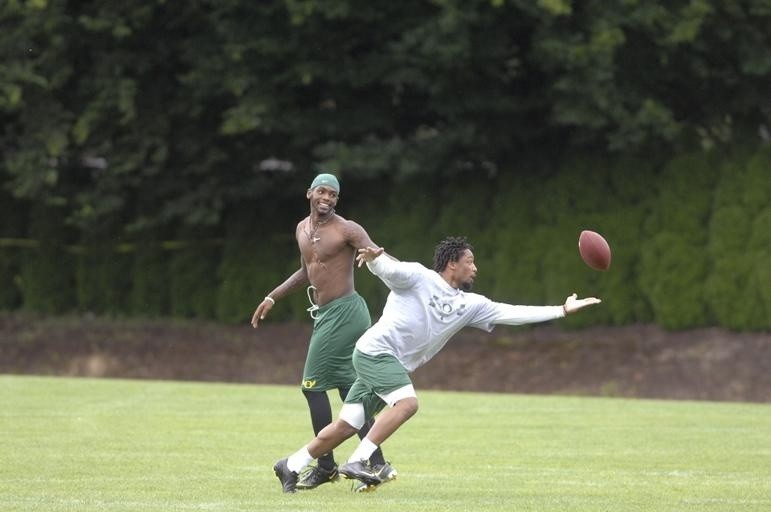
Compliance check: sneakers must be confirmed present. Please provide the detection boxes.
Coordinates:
[354,462,398,494]
[294,462,339,491]
[338,458,382,486]
[272,456,301,498]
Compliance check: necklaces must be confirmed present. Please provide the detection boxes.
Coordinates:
[304,211,337,272]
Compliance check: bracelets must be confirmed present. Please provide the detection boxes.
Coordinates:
[263,292,277,305]
[562,302,572,318]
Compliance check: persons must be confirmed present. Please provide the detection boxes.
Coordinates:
[271,234,604,496]
[250,171,400,494]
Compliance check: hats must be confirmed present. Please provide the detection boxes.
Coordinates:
[310,173,340,193]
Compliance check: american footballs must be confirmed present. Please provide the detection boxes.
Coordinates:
[579,229,610,269]
